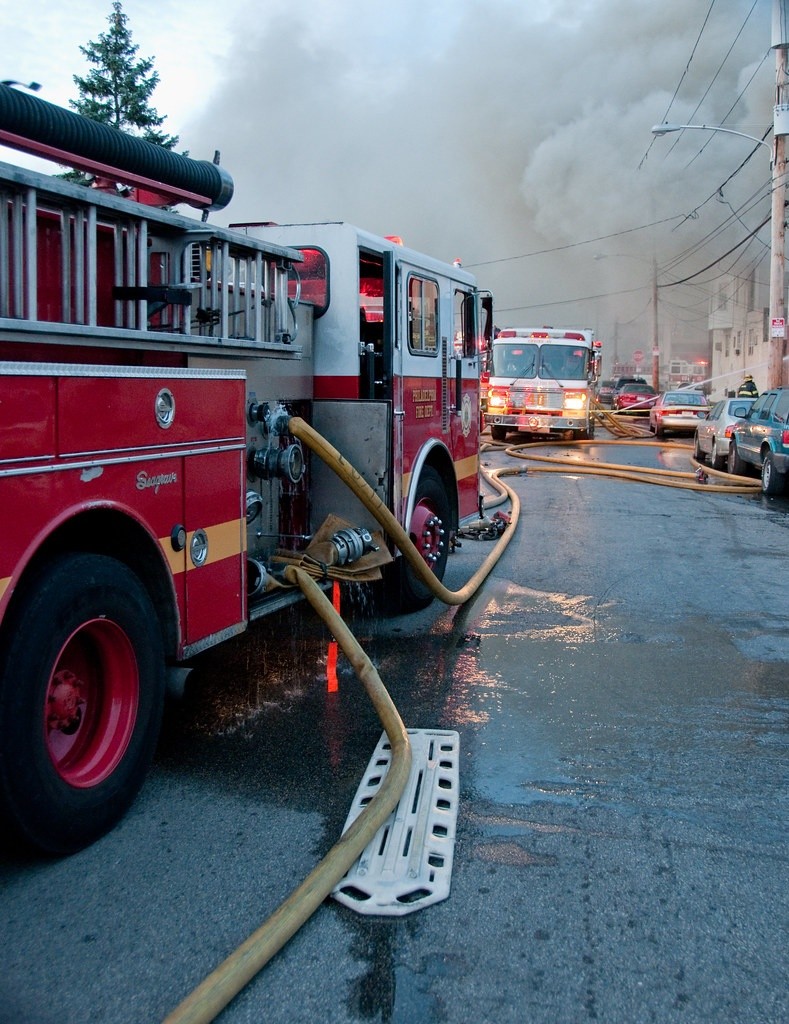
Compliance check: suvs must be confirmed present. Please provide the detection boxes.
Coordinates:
[610,378,648,411]
[728,386,789,496]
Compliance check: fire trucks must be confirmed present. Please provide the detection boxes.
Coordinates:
[486,326,602,441]
[608,359,704,393]
[2,77,487,850]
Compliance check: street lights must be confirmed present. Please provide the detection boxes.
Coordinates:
[651,124,787,394]
[594,253,660,393]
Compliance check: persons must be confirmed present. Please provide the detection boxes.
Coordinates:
[737,373,759,400]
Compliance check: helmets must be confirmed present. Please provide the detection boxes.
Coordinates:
[743,374,754,380]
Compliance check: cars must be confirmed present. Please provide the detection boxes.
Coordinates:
[599,388,618,403]
[694,398,761,469]
[649,391,712,439]
[614,383,659,415]
